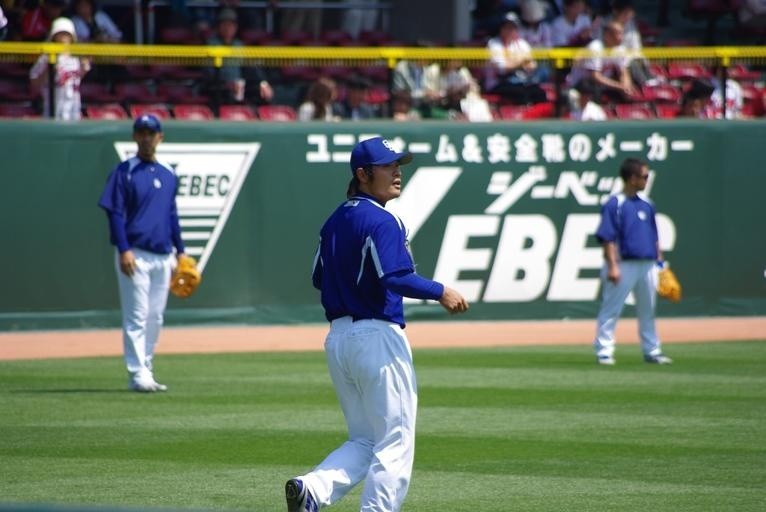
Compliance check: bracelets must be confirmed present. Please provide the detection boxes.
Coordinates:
[259,81,267,86]
[658,261,670,270]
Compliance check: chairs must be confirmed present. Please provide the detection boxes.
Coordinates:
[1,28,766,122]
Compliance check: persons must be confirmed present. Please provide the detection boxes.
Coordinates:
[96,112,202,396]
[17,0,66,41]
[591,157,674,365]
[282,133,472,512]
[202,9,274,104]
[295,2,747,124]
[67,0,124,43]
[27,15,92,120]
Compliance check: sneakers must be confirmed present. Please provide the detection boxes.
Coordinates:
[598,353,674,367]
[285,478,320,511]
[128,375,168,392]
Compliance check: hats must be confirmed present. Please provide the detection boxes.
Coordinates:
[349,137,414,168]
[216,8,240,24]
[132,113,161,134]
[45,16,78,44]
[500,11,522,28]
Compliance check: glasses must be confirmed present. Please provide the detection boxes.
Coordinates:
[636,174,649,178]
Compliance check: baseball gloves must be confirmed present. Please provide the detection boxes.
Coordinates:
[171,255,200,298]
[655,261,681,303]
